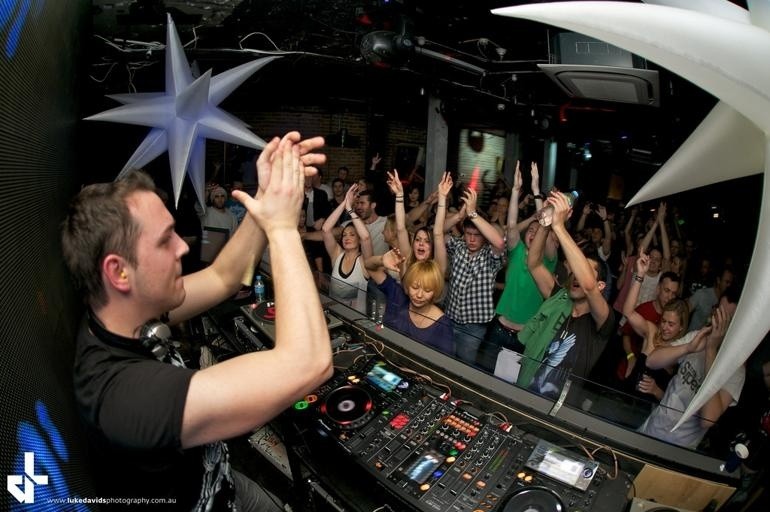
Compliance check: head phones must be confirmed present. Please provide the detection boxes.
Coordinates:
[84,307,171,363]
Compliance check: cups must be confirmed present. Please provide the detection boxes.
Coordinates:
[724,443,749,473]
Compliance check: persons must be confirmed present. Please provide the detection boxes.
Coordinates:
[205,154,746,452]
[59,130,335,511]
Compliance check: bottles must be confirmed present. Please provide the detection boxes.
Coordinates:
[254,276,264,303]
[537,191,578,227]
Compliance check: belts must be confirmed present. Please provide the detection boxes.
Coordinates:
[497,319,518,337]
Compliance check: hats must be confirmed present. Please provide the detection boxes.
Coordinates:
[210,186,228,204]
